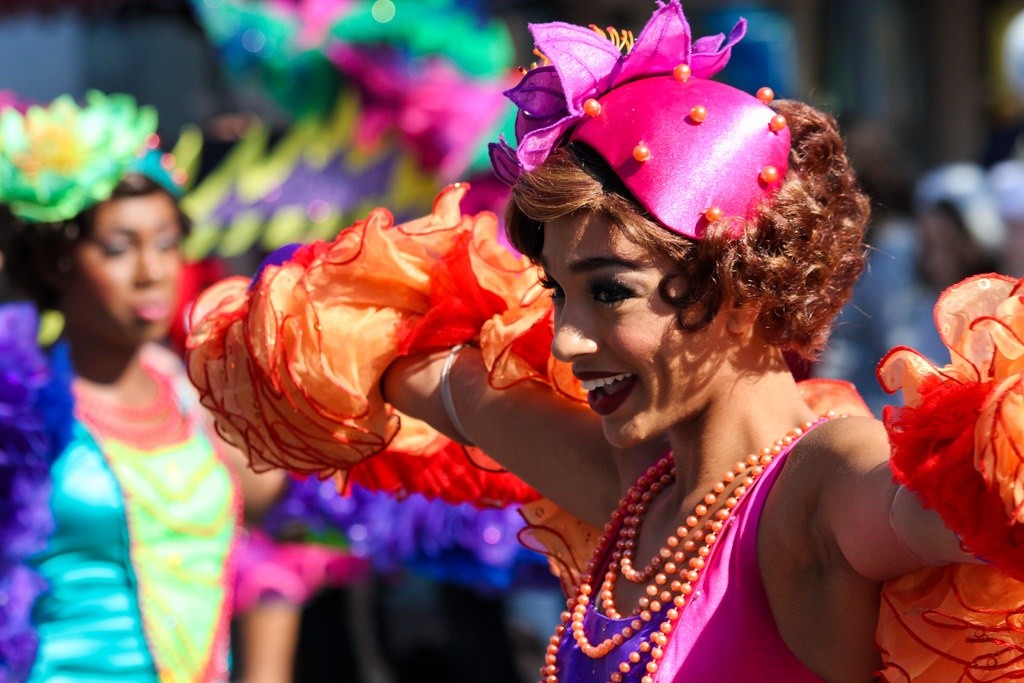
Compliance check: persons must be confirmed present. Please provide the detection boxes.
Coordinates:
[812,118,1024,408]
[0,91,566,683]
[183,1,1024,683]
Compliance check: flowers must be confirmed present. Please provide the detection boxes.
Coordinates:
[0,87,159,223]
[488,0,747,187]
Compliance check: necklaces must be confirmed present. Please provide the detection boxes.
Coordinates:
[546,412,848,683]
